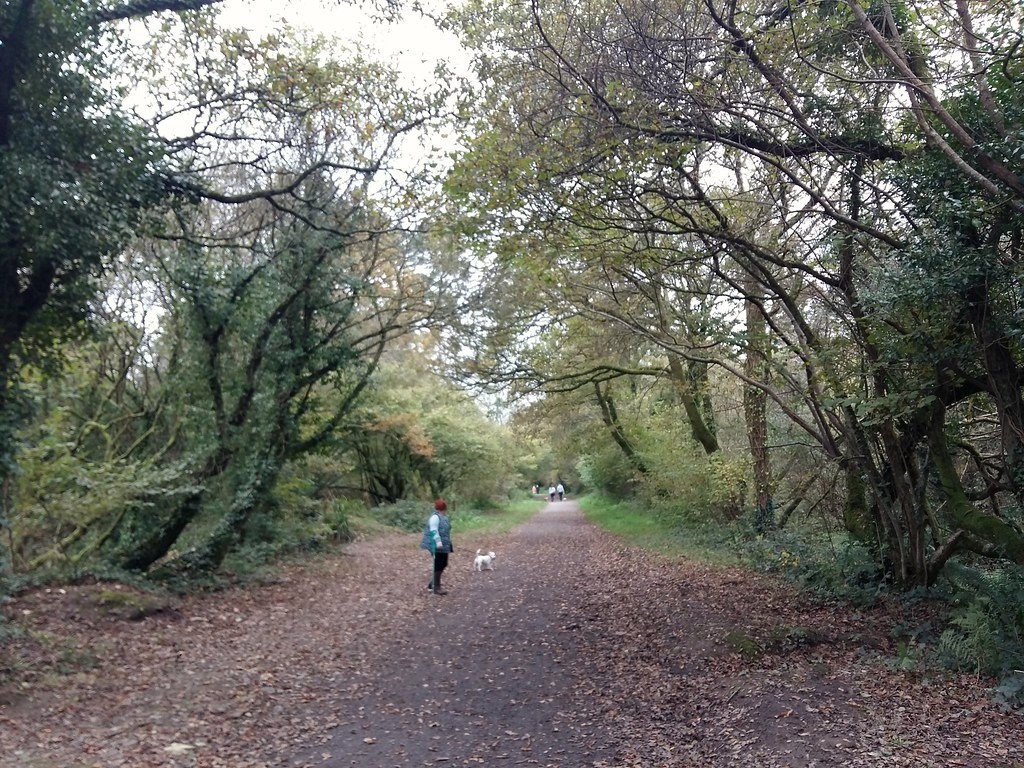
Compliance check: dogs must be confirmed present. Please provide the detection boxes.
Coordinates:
[473,548,497,572]
[563,497,567,501]
[544,496,549,501]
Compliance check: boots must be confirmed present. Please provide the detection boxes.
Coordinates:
[428,571,447,595]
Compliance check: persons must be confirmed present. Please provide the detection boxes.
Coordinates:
[427,501,454,595]
[532,482,566,502]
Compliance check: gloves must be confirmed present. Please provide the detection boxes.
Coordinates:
[436,542,443,548]
